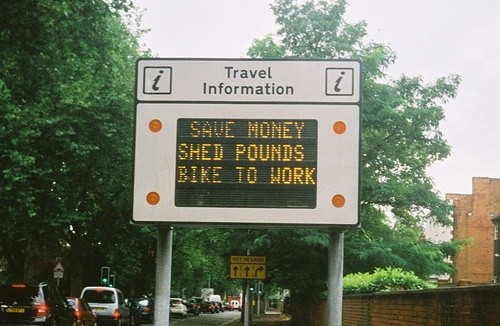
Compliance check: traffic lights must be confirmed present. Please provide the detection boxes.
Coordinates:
[249,281,256,293]
[258,282,263,295]
[100,266,110,285]
[107,275,116,288]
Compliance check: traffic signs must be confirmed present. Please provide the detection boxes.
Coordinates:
[230,256,266,279]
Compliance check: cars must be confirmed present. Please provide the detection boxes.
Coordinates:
[65,297,98,325]
[170,298,188,318]
[0,282,78,326]
[183,298,201,316]
[125,298,154,324]
[201,301,224,313]
[97,303,140,325]
[224,302,235,311]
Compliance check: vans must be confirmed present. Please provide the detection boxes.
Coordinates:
[80,286,125,326]
[192,296,205,306]
